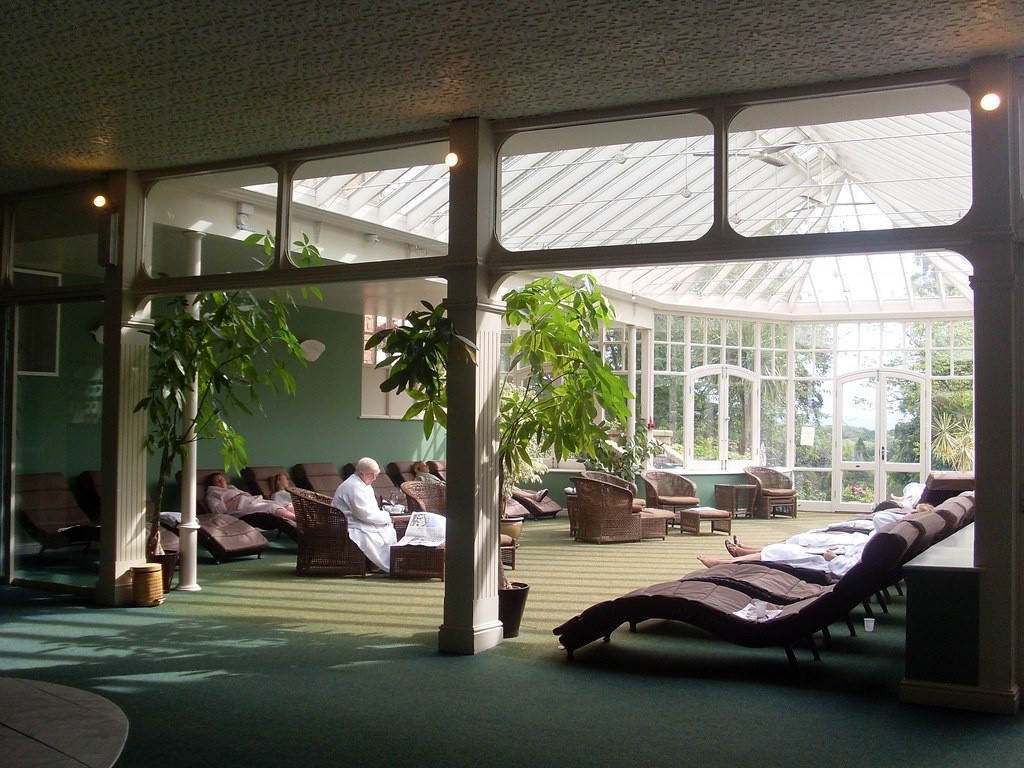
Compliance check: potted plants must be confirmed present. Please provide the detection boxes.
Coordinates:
[59,227,335,592]
[357,270,635,641]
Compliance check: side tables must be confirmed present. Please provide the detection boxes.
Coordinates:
[715,482,758,520]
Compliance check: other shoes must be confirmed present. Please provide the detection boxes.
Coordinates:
[536,489,549,503]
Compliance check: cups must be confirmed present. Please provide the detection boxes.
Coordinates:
[864,618,875,632]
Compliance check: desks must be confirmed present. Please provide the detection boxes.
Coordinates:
[900,520,976,684]
[565,494,579,536]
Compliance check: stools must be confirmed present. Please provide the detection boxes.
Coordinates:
[680,506,730,537]
[390,546,445,580]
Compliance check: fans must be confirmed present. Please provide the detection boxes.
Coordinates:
[791,152,875,212]
[692,132,799,167]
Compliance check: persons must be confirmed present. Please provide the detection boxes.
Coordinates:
[204,471,298,521]
[696,481,934,571]
[330,456,397,572]
[413,459,548,504]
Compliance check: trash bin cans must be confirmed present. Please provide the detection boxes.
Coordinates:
[129,563,165,607]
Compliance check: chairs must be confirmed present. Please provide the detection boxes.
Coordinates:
[553,468,976,661]
[10,464,563,572]
